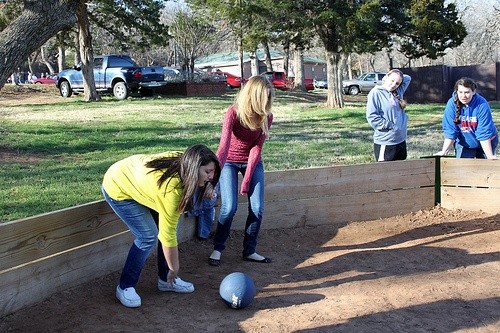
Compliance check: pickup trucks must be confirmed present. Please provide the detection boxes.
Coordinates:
[259,71,315,92]
[56,54,168,100]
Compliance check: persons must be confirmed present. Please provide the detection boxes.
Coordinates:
[103,144,221,306]
[15,70,49,84]
[313,76,319,89]
[202,77,273,265]
[432,77,500,188]
[367,69,411,193]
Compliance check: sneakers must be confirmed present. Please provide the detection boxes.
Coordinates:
[115,284,141,307]
[158,276,195,293]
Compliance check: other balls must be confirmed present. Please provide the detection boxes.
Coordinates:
[219,272,256,310]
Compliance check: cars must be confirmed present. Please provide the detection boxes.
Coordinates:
[33,73,58,85]
[170,66,248,90]
[315,74,328,88]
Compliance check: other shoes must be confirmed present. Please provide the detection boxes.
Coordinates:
[243,252,271,264]
[209,250,219,265]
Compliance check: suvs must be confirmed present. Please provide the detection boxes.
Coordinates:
[342,72,389,95]
